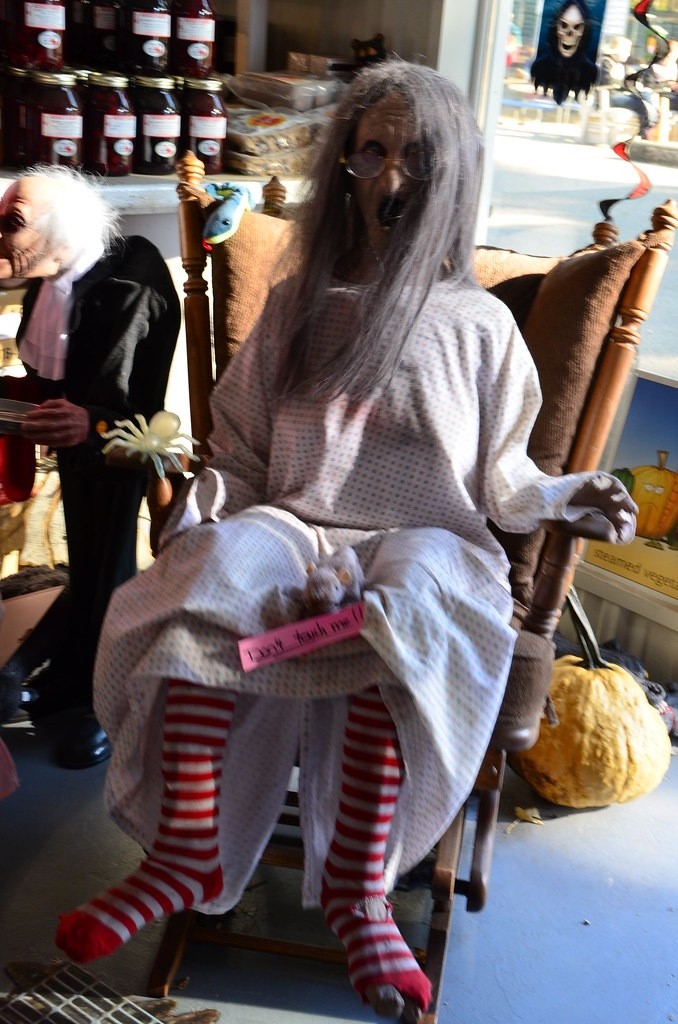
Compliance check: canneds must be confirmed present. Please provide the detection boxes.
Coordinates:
[0,0,225,174]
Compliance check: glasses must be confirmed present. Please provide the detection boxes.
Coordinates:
[337,147,439,181]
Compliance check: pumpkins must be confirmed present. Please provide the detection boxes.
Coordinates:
[504,580,673,808]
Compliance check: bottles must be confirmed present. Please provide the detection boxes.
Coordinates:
[0,0,229,178]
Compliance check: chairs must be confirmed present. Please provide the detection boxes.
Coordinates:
[144,146,678,1024]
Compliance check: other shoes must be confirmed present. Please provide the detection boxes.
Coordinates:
[49,714,112,769]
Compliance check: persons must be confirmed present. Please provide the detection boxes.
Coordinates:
[55,60,639,1024]
[1,160,126,596]
